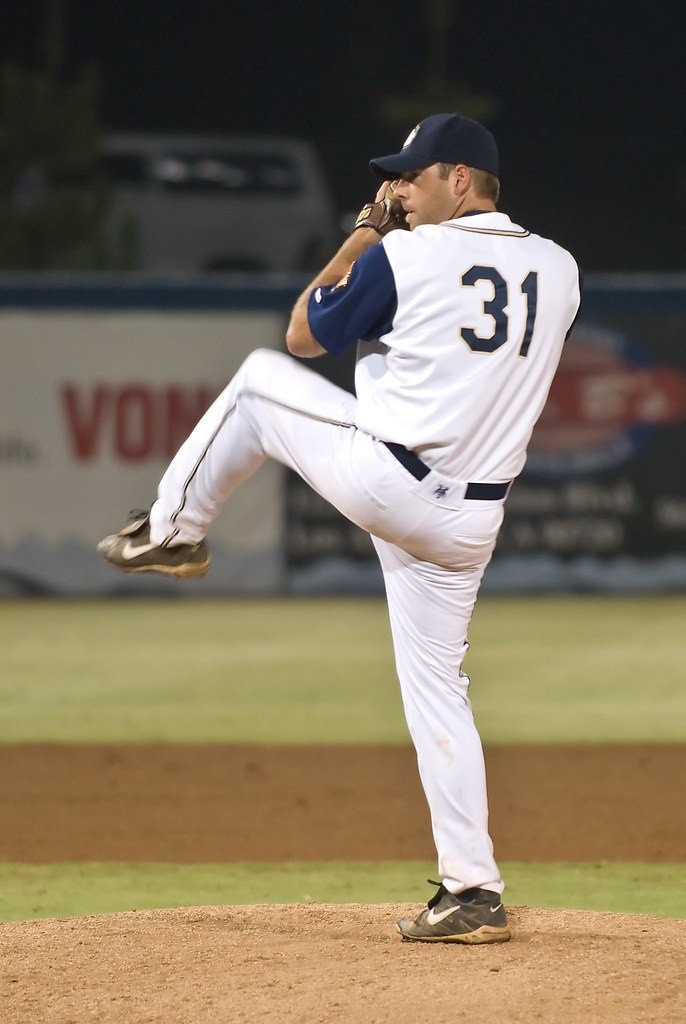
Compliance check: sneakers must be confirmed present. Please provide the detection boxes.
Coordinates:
[396,878,512,943]
[98,509,210,577]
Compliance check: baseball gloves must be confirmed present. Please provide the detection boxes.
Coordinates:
[352,177,412,237]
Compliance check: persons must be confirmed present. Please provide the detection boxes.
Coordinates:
[96,111,581,942]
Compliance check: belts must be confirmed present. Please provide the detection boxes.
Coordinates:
[383,441,510,500]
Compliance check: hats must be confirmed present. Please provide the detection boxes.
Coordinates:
[369,113,499,181]
